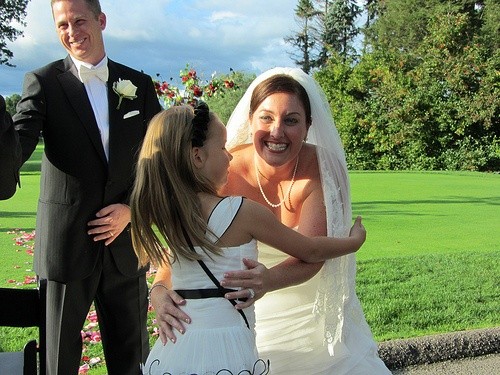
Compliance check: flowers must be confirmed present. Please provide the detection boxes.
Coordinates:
[113,78,137,108]
[152,68,241,112]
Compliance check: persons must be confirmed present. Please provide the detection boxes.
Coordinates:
[0,0,162,375]
[129,100,367,375]
[148,66,391,375]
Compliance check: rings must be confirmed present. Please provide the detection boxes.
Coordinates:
[248,289,255,299]
[109,230,114,237]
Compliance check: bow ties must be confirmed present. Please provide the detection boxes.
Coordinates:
[79,64,108,83]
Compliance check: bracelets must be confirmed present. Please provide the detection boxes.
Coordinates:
[148,284,168,302]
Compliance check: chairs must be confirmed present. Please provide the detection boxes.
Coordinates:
[0,279,47,375]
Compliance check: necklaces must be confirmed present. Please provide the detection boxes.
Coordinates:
[254,148,299,207]
